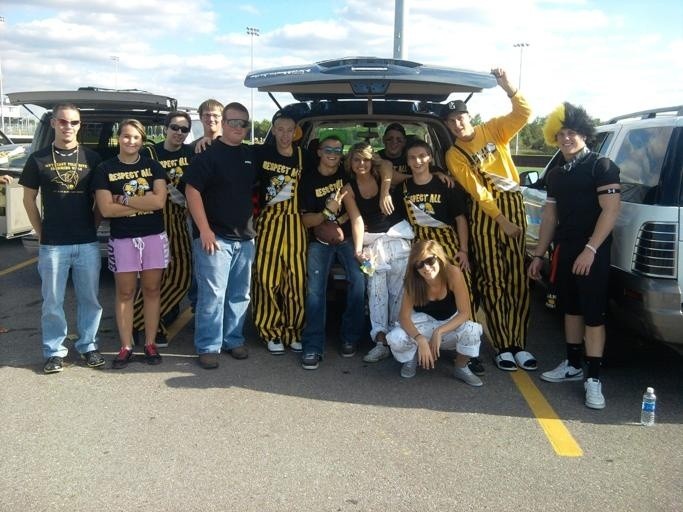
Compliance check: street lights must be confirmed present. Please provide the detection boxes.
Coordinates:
[511,43,529,155]
[243,26,260,144]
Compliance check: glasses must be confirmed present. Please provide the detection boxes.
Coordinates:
[171,124,189,133]
[228,119,248,128]
[323,146,342,155]
[57,118,80,125]
[413,254,436,269]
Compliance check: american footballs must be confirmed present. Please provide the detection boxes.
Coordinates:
[313,222,344,245]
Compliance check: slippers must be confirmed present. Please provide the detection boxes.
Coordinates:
[494,352,518,370]
[514,351,538,369]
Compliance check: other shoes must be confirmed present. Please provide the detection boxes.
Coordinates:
[340,341,356,357]
[302,353,323,369]
[267,340,286,354]
[364,342,390,361]
[199,354,219,369]
[457,365,483,386]
[467,358,485,375]
[230,346,248,359]
[144,344,162,365]
[43,356,62,372]
[112,347,133,368]
[401,354,417,377]
[80,350,106,366]
[289,343,303,352]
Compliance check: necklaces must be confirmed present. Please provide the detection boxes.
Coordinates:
[52,141,79,183]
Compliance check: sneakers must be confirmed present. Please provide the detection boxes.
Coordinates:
[153,326,169,347]
[539,360,584,382]
[584,378,605,408]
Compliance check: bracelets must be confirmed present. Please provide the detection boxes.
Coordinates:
[585,244,596,254]
[533,256,544,260]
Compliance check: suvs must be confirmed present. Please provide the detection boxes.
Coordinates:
[245,57,497,321]
[520,107,683,351]
[3,88,204,262]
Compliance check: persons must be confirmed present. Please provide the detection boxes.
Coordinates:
[526,102,621,410]
[91,99,486,387]
[17,102,106,374]
[445,67,538,371]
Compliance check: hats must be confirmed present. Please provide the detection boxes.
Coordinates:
[272,109,297,124]
[440,100,468,120]
[543,101,594,148]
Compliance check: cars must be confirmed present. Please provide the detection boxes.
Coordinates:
[0,130,32,158]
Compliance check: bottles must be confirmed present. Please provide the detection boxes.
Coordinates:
[641,387,656,426]
